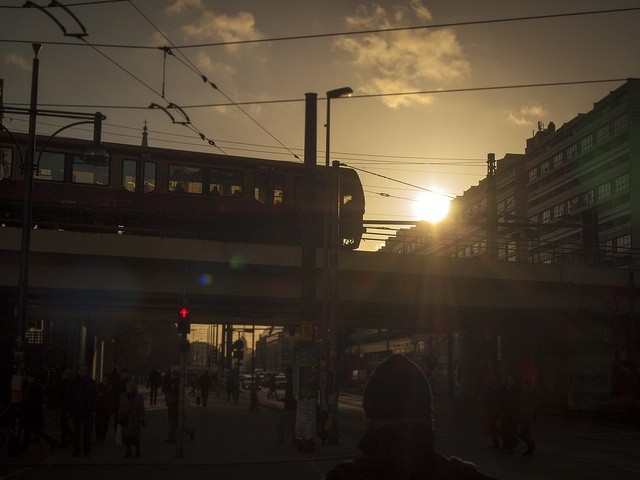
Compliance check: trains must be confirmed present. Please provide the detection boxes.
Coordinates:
[0,132,366,251]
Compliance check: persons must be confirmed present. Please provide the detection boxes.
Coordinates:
[165,369,174,406]
[112,368,128,430]
[117,381,146,458]
[210,186,221,195]
[164,376,196,443]
[487,382,503,448]
[227,370,235,401]
[196,370,212,407]
[146,367,161,406]
[70,366,96,457]
[38,364,54,391]
[175,183,185,192]
[55,369,74,447]
[232,190,240,196]
[274,368,298,446]
[0,364,17,455]
[17,373,57,454]
[108,367,119,381]
[512,379,536,456]
[499,375,520,454]
[325,354,496,480]
[95,376,114,441]
[267,374,279,399]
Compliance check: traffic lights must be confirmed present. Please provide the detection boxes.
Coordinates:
[178,307,191,334]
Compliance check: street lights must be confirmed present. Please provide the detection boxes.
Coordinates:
[325,87,353,167]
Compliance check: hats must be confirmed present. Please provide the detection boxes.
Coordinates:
[363,353,433,421]
